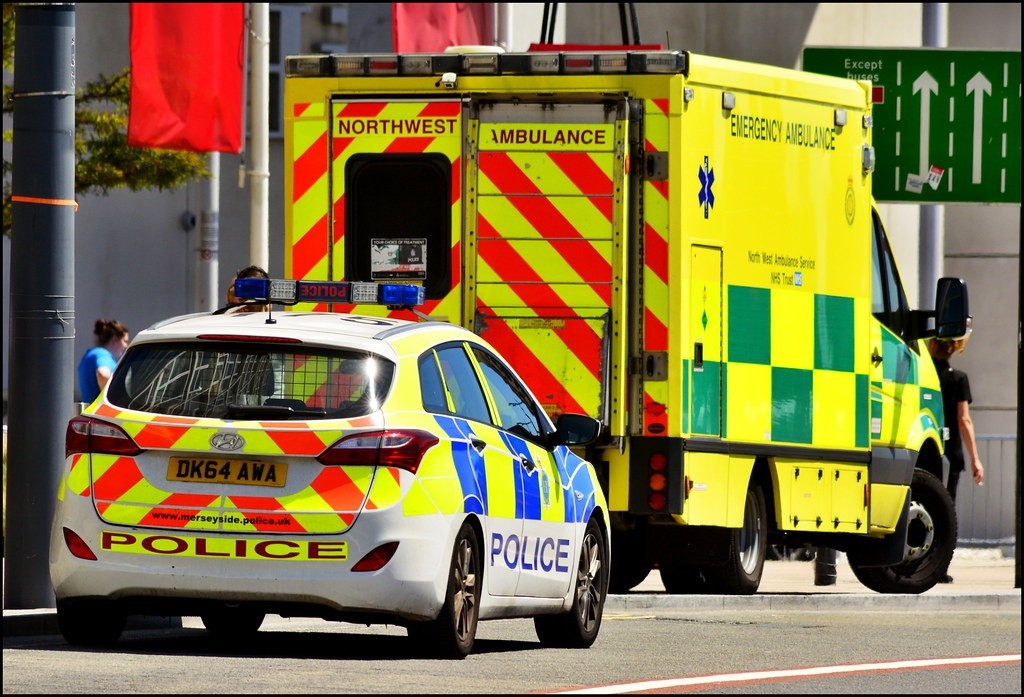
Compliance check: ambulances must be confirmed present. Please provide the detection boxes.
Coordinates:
[279,40,971,598]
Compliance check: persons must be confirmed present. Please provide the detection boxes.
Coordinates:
[929,324,984,583]
[184,266,286,405]
[77,318,129,404]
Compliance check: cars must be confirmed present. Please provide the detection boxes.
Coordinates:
[40,279,616,660]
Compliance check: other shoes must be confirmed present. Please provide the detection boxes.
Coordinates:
[938,574,953,583]
